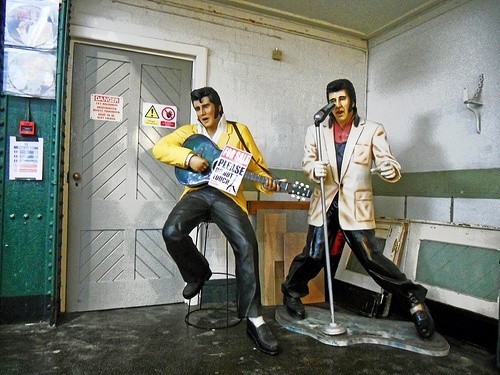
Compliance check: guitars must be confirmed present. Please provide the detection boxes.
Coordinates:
[174,134,313,198]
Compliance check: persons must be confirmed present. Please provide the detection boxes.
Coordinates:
[282,79,435,337]
[153,87,288,353]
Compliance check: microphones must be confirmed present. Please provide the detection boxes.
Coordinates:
[313,103,335,122]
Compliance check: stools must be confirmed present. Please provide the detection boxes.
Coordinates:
[185,218,242,330]
